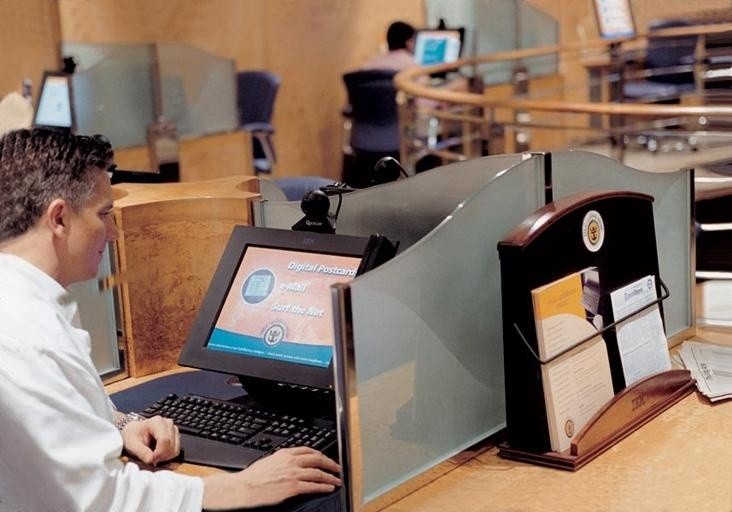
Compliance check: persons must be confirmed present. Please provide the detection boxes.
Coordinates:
[0,127,347,512]
[349,15,443,180]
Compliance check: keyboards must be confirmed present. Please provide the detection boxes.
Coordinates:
[139,391,338,471]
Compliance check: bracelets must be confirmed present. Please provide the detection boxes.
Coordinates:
[115,411,146,431]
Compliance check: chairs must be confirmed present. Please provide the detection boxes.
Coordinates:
[236,68,280,175]
[341,67,403,185]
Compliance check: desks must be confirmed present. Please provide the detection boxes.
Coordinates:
[102,328,730,512]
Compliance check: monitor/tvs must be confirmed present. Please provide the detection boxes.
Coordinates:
[593,0,636,39]
[32,70,72,132]
[413,28,464,77]
[176,223,395,392]
